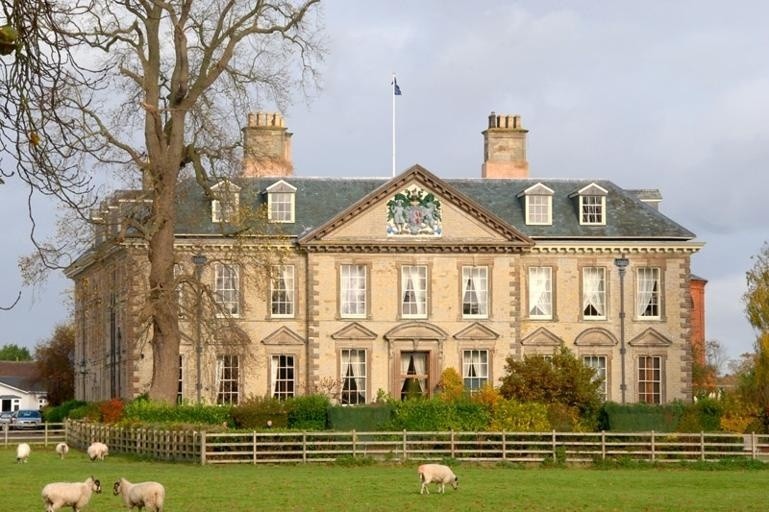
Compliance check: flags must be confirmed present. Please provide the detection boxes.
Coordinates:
[391,77,402,95]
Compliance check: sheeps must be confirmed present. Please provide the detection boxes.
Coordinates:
[41,475,102,512]
[418,463,461,495]
[114,476,165,512]
[86,441,109,463]
[56,441,70,460]
[16,442,31,464]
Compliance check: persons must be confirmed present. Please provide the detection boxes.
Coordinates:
[390,201,409,235]
[421,203,442,230]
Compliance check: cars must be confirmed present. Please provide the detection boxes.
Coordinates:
[12,408,41,433]
[0,411,16,431]
